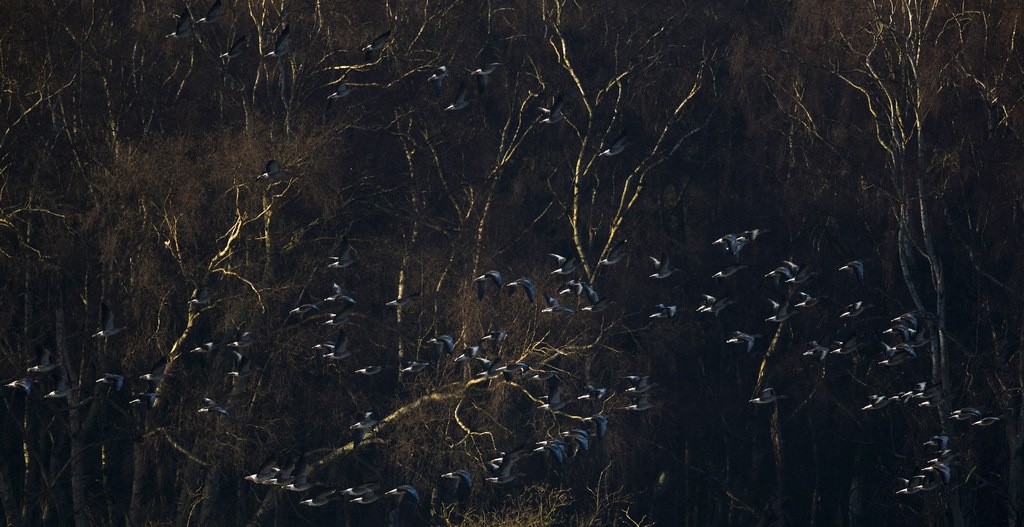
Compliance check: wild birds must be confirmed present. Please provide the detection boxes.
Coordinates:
[537,96,566,124]
[166,0,290,60]
[599,134,636,156]
[255,158,289,181]
[649,249,679,279]
[428,62,500,111]
[327,79,354,98]
[4,234,658,513]
[361,29,391,52]
[648,302,677,318]
[696,226,1003,502]
